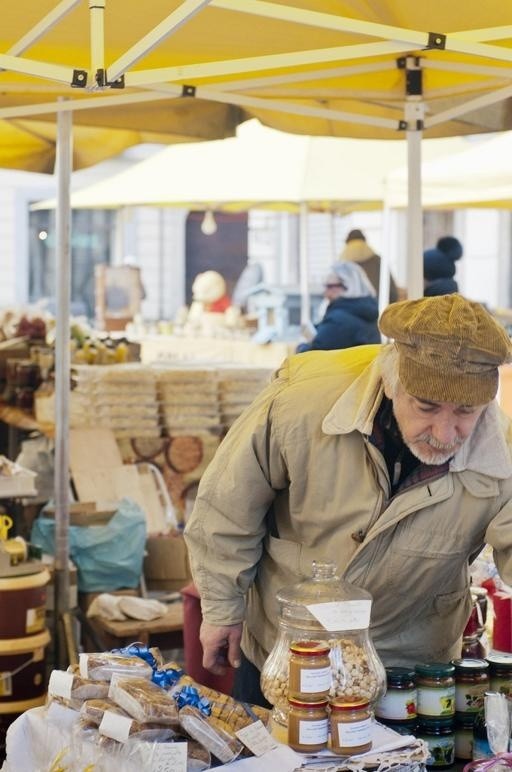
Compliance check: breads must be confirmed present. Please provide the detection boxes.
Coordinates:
[44,647,268,761]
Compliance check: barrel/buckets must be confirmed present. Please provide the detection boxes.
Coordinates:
[1,695,45,767]
[0,567,52,640]
[0,628,52,703]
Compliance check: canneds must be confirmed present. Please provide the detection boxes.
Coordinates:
[450,658,489,727]
[456,723,474,759]
[289,641,332,701]
[486,655,512,699]
[287,696,330,751]
[328,697,372,755]
[421,727,456,768]
[375,667,418,734]
[415,661,455,735]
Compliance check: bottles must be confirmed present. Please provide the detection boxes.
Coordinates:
[288,641,511,764]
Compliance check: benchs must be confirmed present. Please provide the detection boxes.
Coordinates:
[92,601,185,646]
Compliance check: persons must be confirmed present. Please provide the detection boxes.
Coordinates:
[288,262,379,354]
[184,291,511,770]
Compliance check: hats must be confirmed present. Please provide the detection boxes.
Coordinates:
[379,292,512,404]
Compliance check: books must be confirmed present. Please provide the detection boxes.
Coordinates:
[299,318,318,343]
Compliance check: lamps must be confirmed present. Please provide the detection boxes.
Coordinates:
[201,211,216,235]
